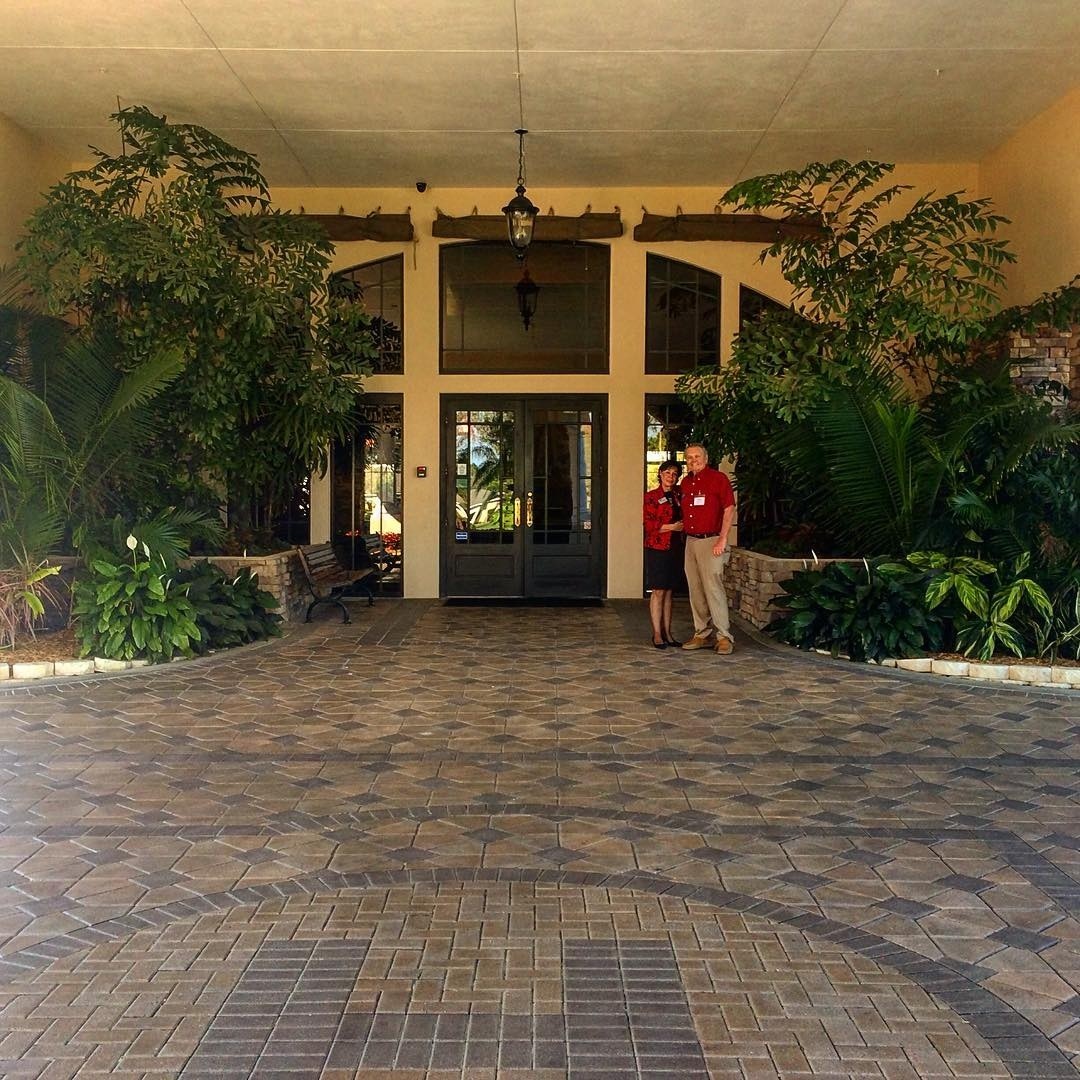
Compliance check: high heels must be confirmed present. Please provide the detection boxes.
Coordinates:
[653,635,666,650]
[662,633,682,647]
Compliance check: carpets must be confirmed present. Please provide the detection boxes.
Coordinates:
[442,598,606,608]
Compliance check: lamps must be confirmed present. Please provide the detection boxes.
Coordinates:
[513,269,541,333]
[502,130,539,271]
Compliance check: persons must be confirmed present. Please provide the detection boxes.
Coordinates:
[678,443,735,654]
[643,460,682,650]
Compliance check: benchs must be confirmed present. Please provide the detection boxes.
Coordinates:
[297,540,377,623]
[357,532,401,580]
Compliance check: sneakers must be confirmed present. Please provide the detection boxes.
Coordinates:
[682,636,714,650]
[714,636,732,654]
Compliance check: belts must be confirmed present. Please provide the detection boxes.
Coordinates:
[690,532,719,540]
